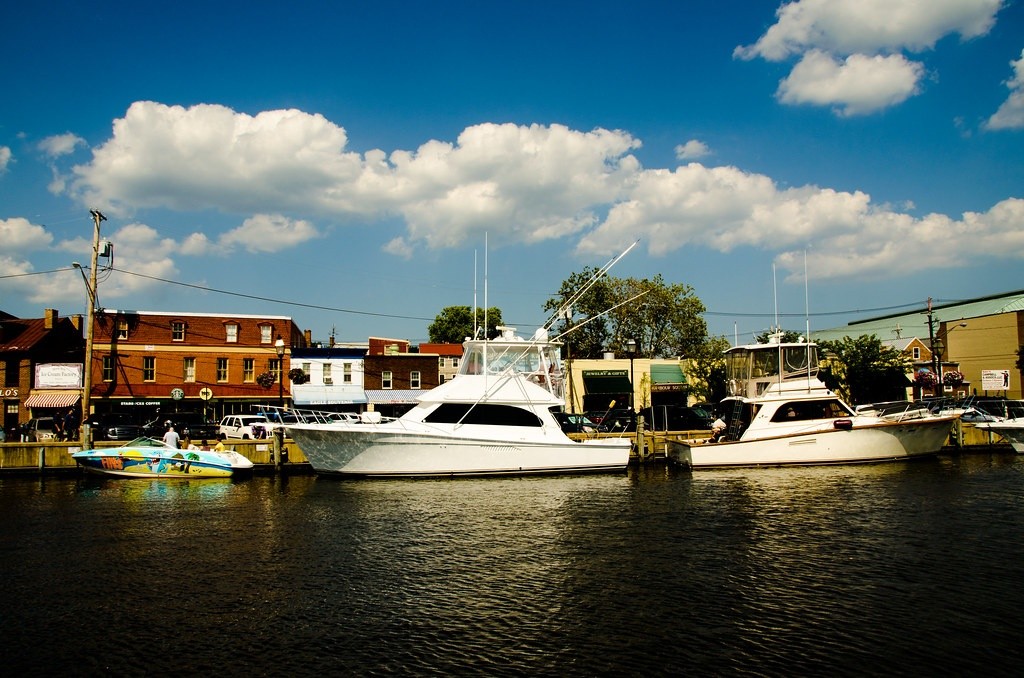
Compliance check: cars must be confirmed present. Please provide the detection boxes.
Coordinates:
[83,412,143,440]
[956,396,1009,419]
[219,415,273,440]
[142,412,202,438]
[258,412,360,424]
[26,416,60,442]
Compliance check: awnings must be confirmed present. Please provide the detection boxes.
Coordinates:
[293,386,367,405]
[364,390,432,404]
[582,369,635,395]
[23,394,80,407]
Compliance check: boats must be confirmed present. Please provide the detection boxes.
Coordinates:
[667,250,975,465]
[278,229,650,473]
[975,416,1024,453]
[71,436,255,479]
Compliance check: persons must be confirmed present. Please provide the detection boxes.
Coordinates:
[1001,371,1009,388]
[52,408,65,442]
[63,408,75,442]
[162,427,225,451]
[708,409,728,443]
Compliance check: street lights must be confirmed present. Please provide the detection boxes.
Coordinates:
[274,334,286,413]
[933,338,945,397]
[72,261,97,450]
[930,323,968,395]
